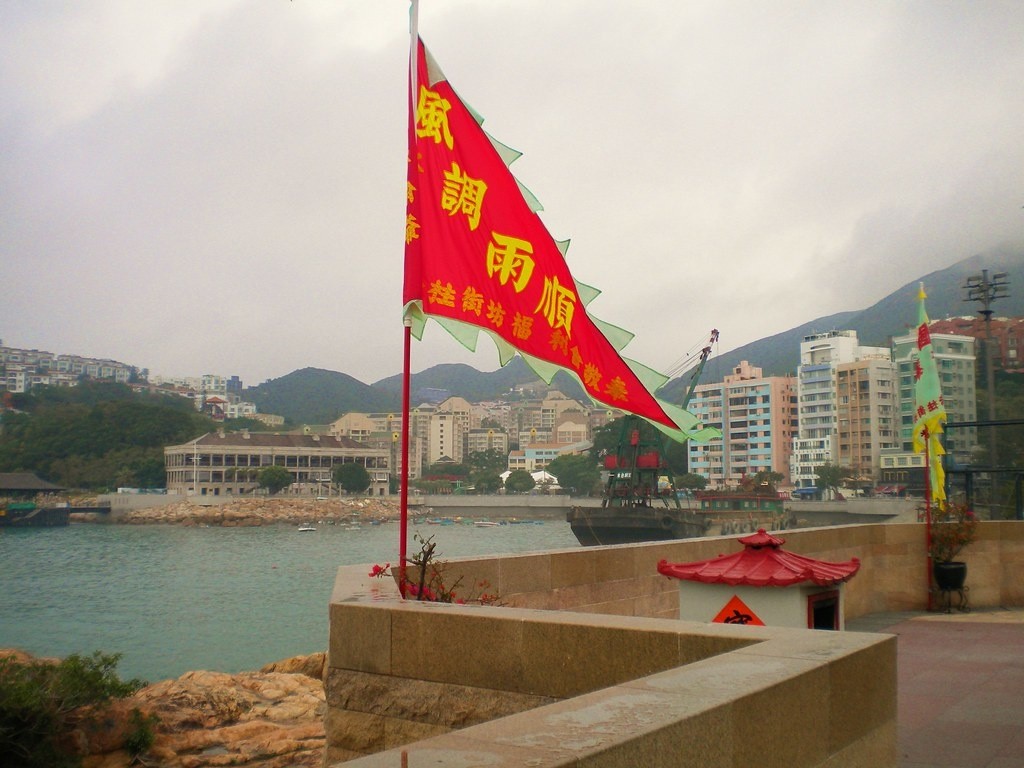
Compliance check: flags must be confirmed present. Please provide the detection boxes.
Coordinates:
[402,29,723,443]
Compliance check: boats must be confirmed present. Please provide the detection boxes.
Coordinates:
[565,328,799,548]
[293,510,546,533]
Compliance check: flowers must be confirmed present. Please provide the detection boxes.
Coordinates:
[915,496,983,564]
[367,531,525,610]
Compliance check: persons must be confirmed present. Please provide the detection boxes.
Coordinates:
[912,299,946,511]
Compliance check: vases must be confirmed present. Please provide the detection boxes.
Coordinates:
[933,560,968,594]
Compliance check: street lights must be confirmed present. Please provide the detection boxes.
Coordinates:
[961,268,1014,518]
